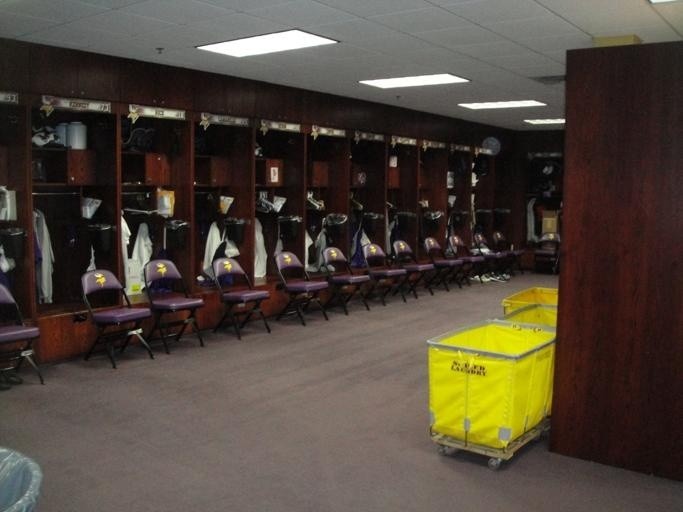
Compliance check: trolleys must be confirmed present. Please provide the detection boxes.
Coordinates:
[501,287,557,326]
[423,319,556,472]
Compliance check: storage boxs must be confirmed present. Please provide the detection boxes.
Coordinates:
[591,34,641,47]
[426,318,554,449]
[488,304,558,335]
[496,284,559,325]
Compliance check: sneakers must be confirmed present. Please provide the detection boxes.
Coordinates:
[468,271,511,283]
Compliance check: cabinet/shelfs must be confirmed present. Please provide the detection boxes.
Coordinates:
[0,88,31,328]
[548,42,683,483]
[32,89,120,332]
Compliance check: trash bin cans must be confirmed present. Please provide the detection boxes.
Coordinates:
[0,446,41,512]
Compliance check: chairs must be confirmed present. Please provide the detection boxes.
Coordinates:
[265,248,334,327]
[76,269,157,370]
[0,279,44,389]
[317,241,370,318]
[199,258,277,340]
[140,260,206,355]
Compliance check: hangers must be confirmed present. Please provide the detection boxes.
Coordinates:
[32,196,43,216]
